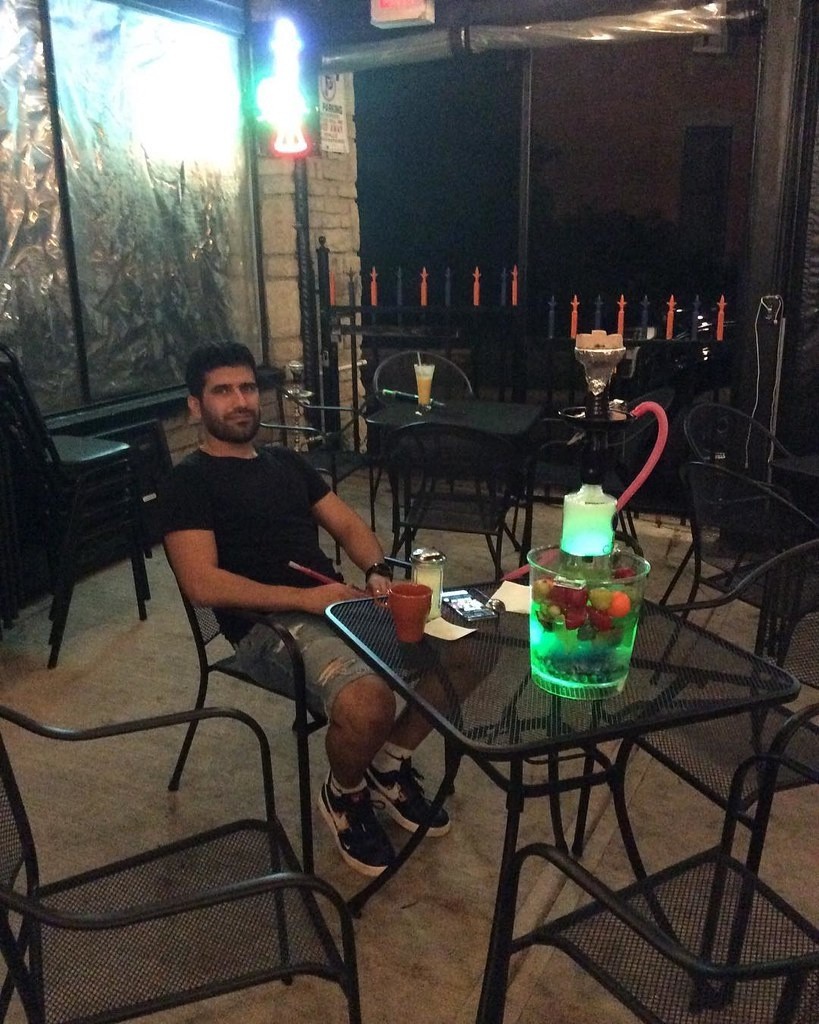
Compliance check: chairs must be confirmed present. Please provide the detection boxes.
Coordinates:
[0,704,362,1024]
[136,350,819,1023]
[0,341,153,669]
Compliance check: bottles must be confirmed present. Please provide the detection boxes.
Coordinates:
[409,544,446,622]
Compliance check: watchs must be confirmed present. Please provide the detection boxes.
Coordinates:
[365,563,393,582]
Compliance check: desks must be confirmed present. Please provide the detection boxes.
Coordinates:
[769,455,819,480]
[366,400,544,577]
[326,575,802,1023]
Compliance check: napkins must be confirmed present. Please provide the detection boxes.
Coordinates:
[485,580,530,615]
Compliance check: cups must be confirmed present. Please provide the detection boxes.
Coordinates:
[414,363,436,412]
[374,583,433,643]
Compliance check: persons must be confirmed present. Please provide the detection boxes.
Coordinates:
[159,342,452,877]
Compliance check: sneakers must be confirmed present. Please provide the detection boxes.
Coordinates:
[317,768,395,877]
[362,758,451,837]
